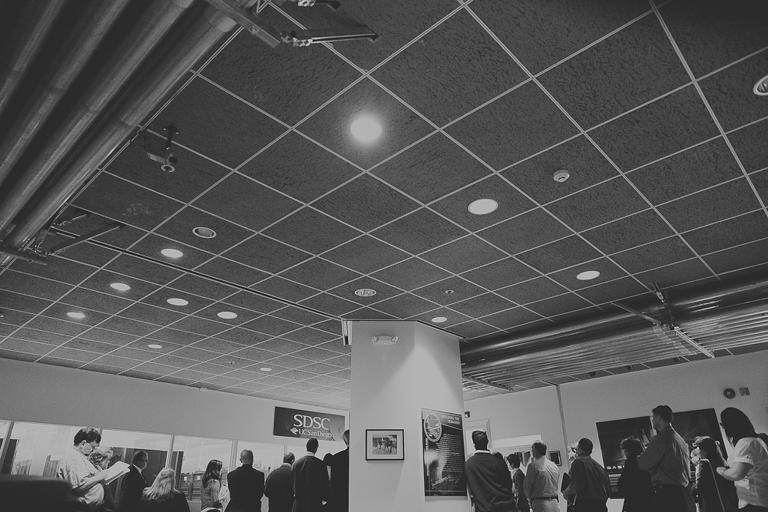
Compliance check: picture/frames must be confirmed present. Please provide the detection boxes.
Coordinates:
[547,450,562,466]
[365,428,404,460]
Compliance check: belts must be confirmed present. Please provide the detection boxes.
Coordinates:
[534,496,560,501]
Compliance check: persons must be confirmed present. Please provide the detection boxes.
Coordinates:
[57,429,349,512]
